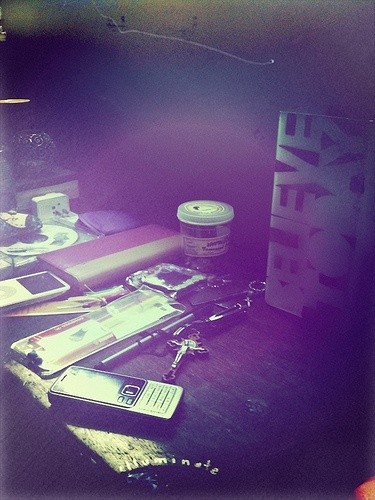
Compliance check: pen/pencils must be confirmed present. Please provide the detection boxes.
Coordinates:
[95,313,197,367]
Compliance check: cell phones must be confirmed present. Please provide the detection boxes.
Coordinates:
[48,364,186,422]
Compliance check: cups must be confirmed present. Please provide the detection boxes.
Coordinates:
[176,200,235,277]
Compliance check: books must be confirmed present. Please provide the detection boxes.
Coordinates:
[37,222,182,293]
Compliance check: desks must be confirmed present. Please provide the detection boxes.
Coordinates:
[2,202,374,500]
